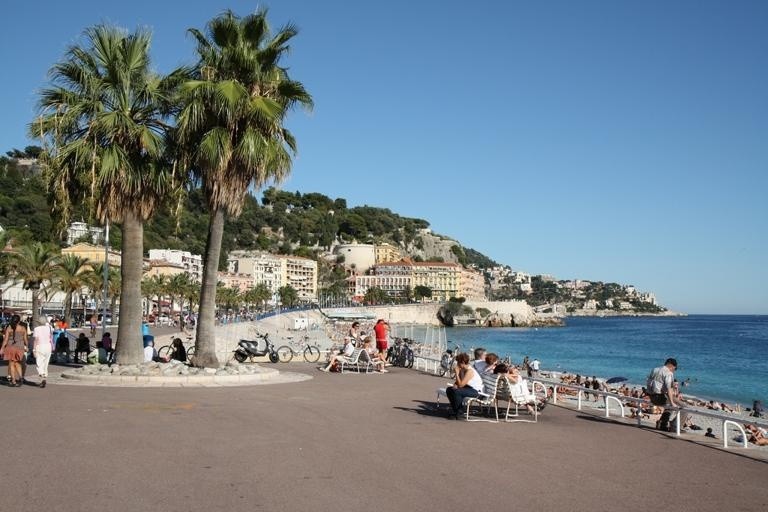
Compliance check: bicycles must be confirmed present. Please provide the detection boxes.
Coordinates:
[158,335,196,361]
[386,336,415,369]
[439,340,459,378]
[276,335,320,364]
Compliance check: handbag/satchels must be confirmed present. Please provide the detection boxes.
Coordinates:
[649,392,667,406]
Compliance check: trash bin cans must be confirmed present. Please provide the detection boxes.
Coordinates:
[143,324,149,336]
[53,330,66,345]
[295,318,307,329]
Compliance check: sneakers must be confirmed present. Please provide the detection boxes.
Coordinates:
[7,378,47,388]
[669,421,687,433]
[379,369,390,373]
[526,396,548,416]
[656,420,670,431]
[384,363,394,366]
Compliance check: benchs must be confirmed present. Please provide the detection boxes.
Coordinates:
[28,350,96,363]
[436,370,548,423]
[335,346,384,374]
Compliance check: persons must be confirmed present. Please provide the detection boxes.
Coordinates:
[446,352,483,420]
[483,353,498,372]
[492,363,550,417]
[646,358,689,432]
[705,398,768,440]
[522,355,663,423]
[0,365,54,388]
[471,348,488,371]
[673,378,679,396]
[676,390,682,399]
[319,317,393,373]
[0,303,266,366]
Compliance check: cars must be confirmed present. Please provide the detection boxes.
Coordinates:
[1,308,187,334]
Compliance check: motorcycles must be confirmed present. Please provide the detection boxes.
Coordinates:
[233,332,278,365]
